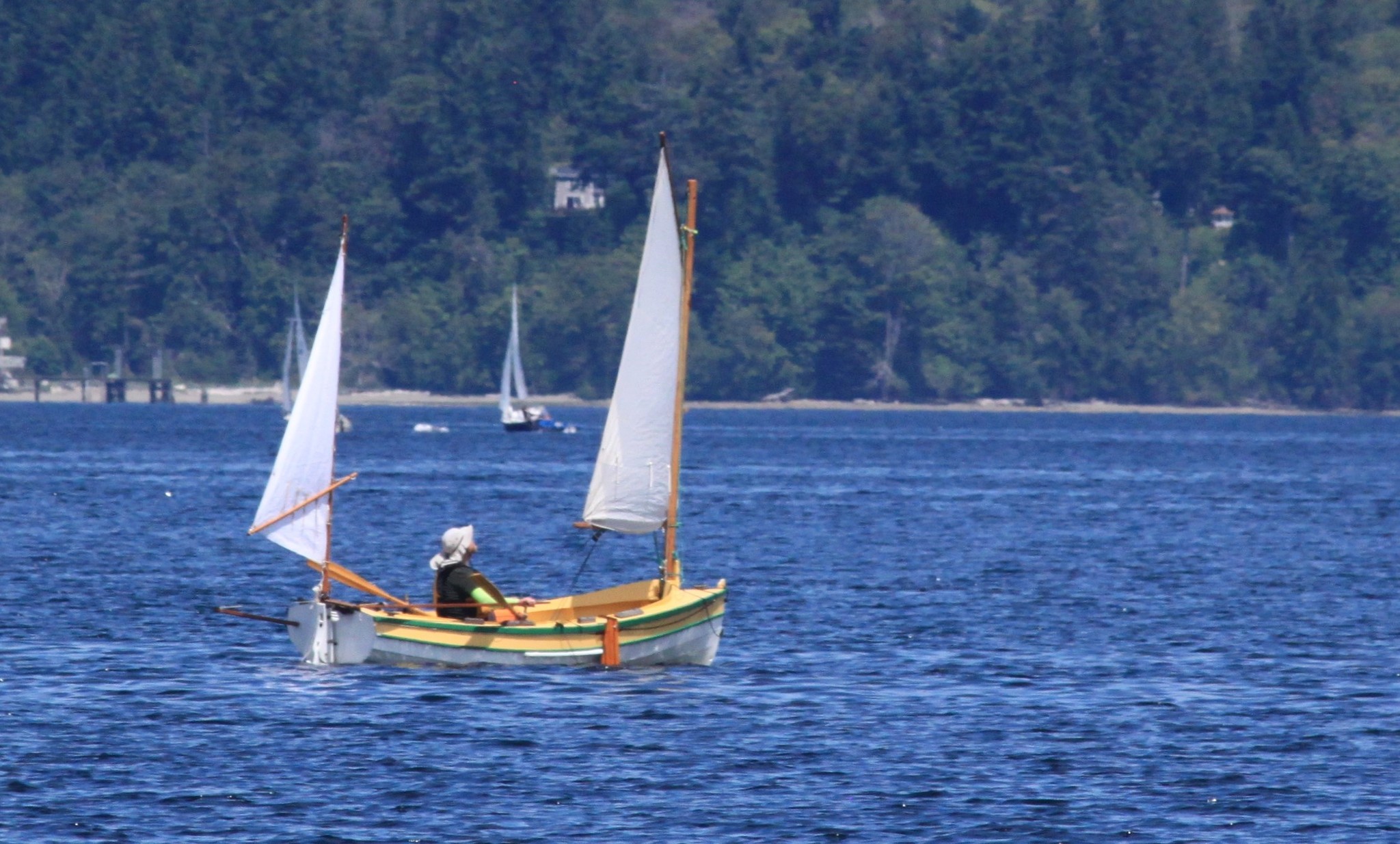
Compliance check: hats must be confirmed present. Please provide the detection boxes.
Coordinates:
[428,525,473,569]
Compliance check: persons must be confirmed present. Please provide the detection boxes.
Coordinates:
[429,525,538,625]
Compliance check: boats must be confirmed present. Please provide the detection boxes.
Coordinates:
[413,423,452,434]
[535,418,579,433]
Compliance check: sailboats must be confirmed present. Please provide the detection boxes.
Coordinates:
[214,131,727,675]
[280,286,355,435]
[498,283,554,433]
